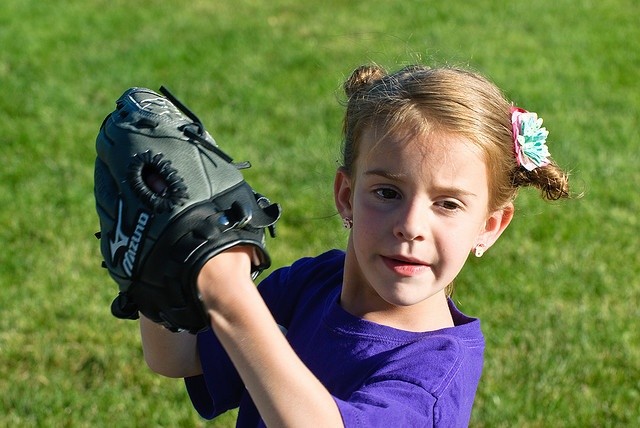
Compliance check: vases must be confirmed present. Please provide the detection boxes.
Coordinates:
[511,109,552,172]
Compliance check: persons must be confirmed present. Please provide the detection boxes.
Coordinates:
[93,62,572,427]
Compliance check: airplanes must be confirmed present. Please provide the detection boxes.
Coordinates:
[94,86,281,336]
